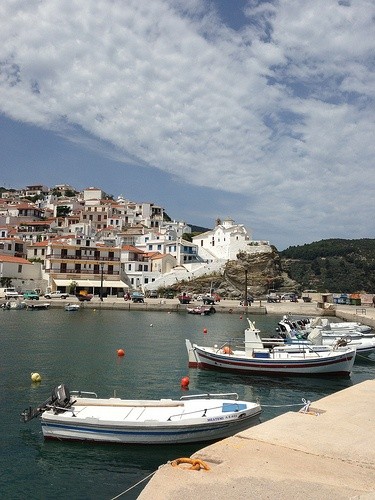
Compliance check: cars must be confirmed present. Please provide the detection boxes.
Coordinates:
[23,290,39,300]
[163,292,222,305]
[239,294,254,303]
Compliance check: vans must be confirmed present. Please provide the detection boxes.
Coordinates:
[281,293,295,300]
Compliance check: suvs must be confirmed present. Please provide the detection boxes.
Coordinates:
[1,288,19,300]
[150,291,158,298]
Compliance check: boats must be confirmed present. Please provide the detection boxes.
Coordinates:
[275,314,375,360]
[41,392,263,446]
[65,303,80,311]
[1,301,50,311]
[185,318,357,379]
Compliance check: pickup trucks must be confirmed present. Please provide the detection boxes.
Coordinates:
[44,290,69,299]
